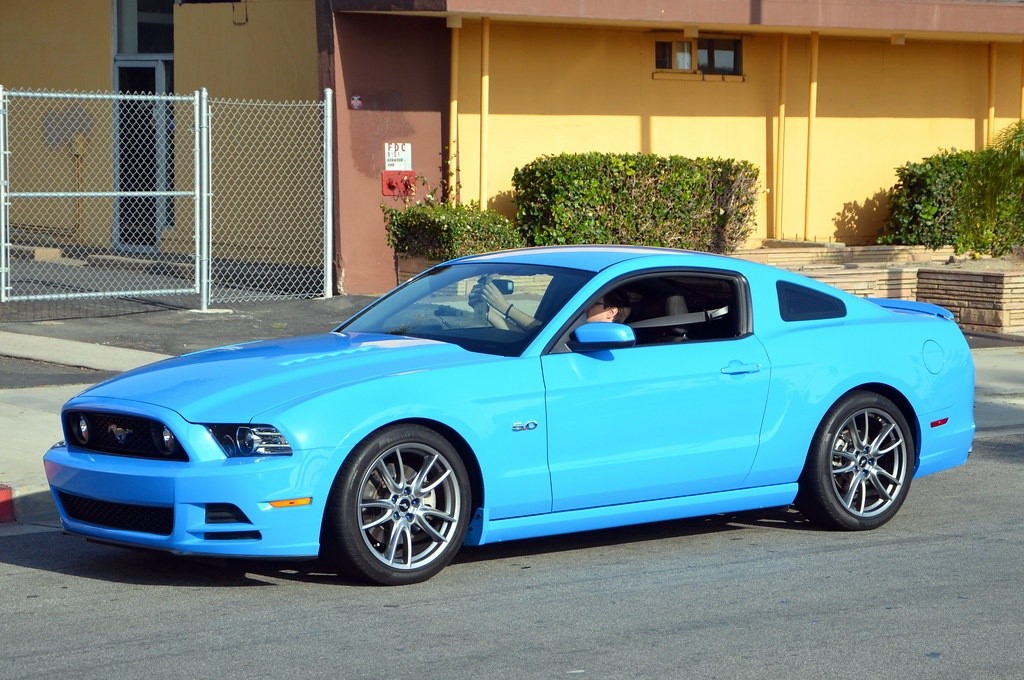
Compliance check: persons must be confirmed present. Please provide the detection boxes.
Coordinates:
[468,281,633,337]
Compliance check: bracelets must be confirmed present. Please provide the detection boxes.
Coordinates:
[505,304,514,319]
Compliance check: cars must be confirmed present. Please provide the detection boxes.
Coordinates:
[42,244,976,583]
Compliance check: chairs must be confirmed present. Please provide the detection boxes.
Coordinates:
[633,293,689,344]
